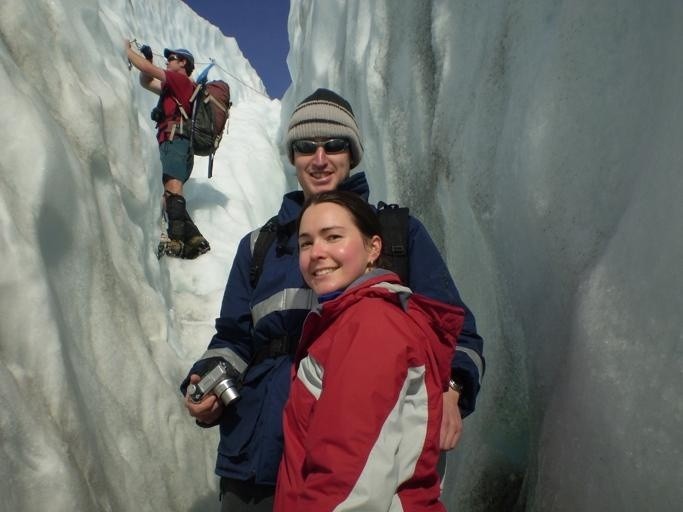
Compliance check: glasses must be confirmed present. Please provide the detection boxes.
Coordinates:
[290,139,349,155]
[167,56,183,61]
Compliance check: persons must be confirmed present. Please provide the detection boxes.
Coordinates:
[125,36,211,260]
[271,189,467,512]
[177,83,489,512]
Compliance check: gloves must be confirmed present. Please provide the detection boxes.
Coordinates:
[140,44,154,59]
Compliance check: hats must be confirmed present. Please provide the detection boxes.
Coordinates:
[285,88,363,169]
[163,47,195,72]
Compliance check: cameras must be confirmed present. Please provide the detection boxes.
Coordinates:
[150,107,165,122]
[187,359,241,407]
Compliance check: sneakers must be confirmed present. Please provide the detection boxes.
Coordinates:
[157,233,211,260]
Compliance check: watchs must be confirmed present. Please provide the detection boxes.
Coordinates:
[447,372,465,397]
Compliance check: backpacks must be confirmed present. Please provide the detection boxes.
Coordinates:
[190,79,230,157]
